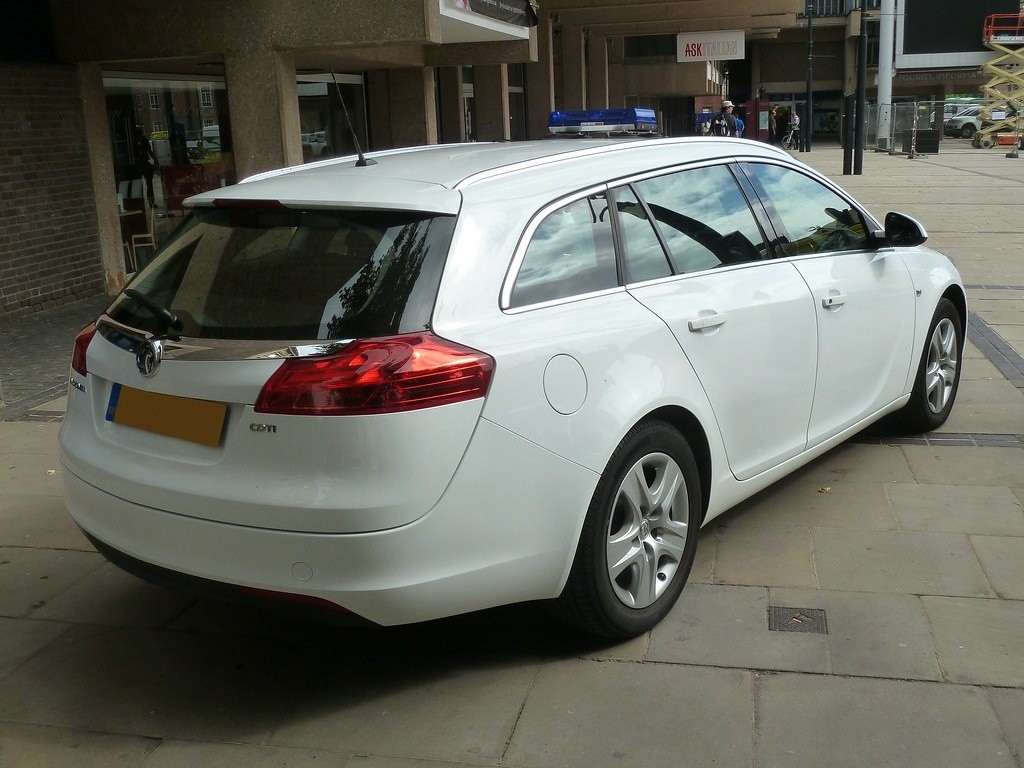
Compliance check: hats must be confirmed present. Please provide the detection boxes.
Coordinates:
[720,101,736,109]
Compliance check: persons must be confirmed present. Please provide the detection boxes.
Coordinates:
[699,118,711,135]
[735,114,745,137]
[769,107,779,145]
[788,111,800,150]
[708,101,738,138]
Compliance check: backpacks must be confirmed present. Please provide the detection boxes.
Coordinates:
[710,114,732,137]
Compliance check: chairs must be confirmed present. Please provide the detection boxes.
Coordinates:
[123,241,134,273]
[131,207,158,272]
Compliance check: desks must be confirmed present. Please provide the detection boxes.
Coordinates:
[118,210,142,216]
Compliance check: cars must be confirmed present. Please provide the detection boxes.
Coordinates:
[301,134,328,156]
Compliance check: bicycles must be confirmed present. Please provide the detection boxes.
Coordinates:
[781,128,796,151]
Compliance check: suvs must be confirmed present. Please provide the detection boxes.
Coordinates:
[57,108,967,643]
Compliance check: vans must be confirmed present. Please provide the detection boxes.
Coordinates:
[930,104,1014,139]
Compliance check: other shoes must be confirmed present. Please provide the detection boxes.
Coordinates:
[794,148,798,150]
